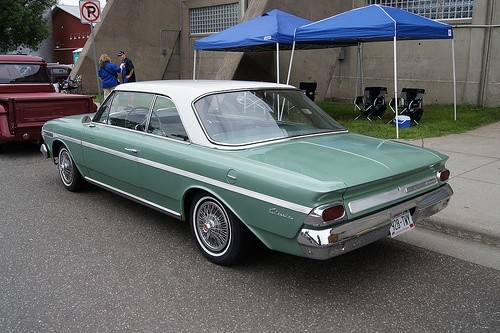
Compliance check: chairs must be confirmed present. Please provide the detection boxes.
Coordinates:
[15,67,33,81]
[353,86,388,122]
[388,88,425,125]
[288,82,317,114]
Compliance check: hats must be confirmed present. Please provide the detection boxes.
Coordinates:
[117,51,125,55]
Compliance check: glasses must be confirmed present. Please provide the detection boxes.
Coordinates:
[118,55,122,57]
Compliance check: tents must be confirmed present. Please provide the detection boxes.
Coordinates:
[280,4,456,138]
[193,9,365,120]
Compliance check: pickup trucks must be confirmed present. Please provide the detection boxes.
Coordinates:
[0,54,98,156]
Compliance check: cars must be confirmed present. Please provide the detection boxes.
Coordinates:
[40,79,455,268]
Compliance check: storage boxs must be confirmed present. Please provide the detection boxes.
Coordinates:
[394,114,411,129]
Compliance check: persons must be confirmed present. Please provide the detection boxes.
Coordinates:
[98,54,124,113]
[116,51,136,110]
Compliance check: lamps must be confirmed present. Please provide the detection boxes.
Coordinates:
[55,32,90,49]
